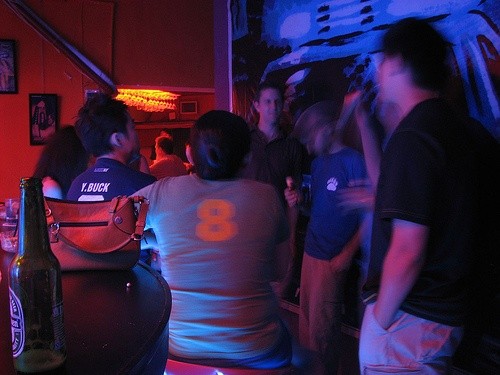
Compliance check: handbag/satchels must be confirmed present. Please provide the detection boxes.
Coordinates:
[43,195,150,272]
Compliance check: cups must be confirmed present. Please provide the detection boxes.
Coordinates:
[0,199,20,252]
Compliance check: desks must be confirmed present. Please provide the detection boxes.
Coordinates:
[0,218,172,375]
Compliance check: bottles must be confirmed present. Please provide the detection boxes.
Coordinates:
[8,177,66,372]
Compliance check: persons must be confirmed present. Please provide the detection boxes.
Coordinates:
[124,110,293,375]
[241,84,376,375]
[30,93,158,203]
[148,136,188,180]
[359,17,496,375]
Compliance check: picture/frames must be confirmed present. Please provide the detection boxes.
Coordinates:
[0,39,18,94]
[28,93,58,145]
